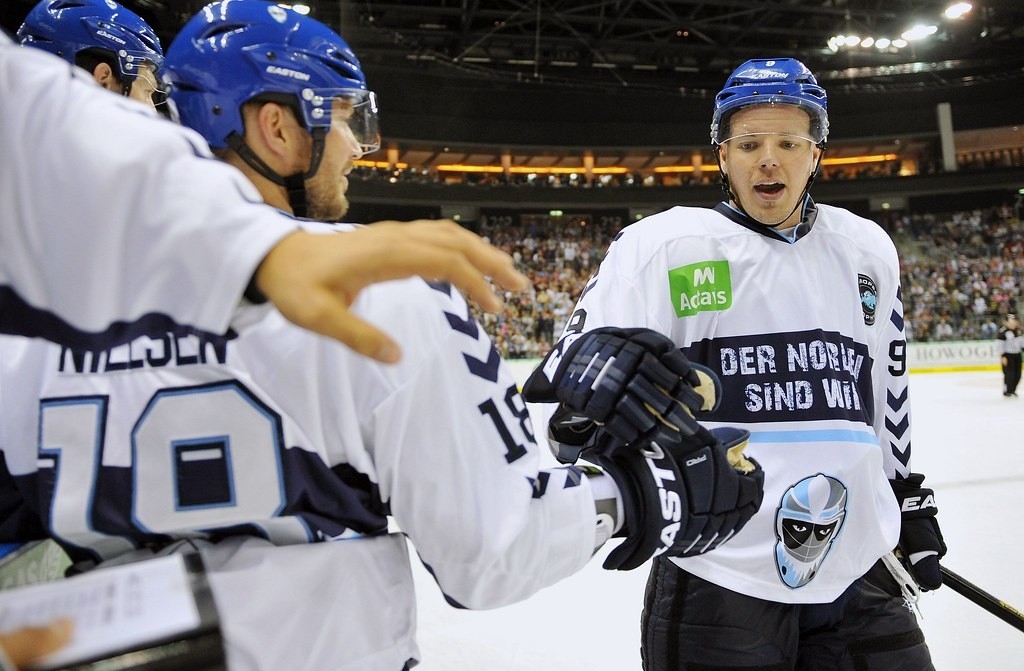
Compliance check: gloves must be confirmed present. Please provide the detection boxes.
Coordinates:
[888,472,947,593]
[518,326,714,451]
[579,425,766,571]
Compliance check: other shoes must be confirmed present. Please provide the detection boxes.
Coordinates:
[1003,392,1020,400]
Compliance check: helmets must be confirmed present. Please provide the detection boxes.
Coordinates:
[710,57,830,181]
[15,0,168,107]
[160,0,381,185]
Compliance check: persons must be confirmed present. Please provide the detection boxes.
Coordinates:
[346,147,1024,358]
[992,313,1023,399]
[11,0,171,117]
[0,1,766,670]
[519,57,947,670]
[0,35,525,670]
